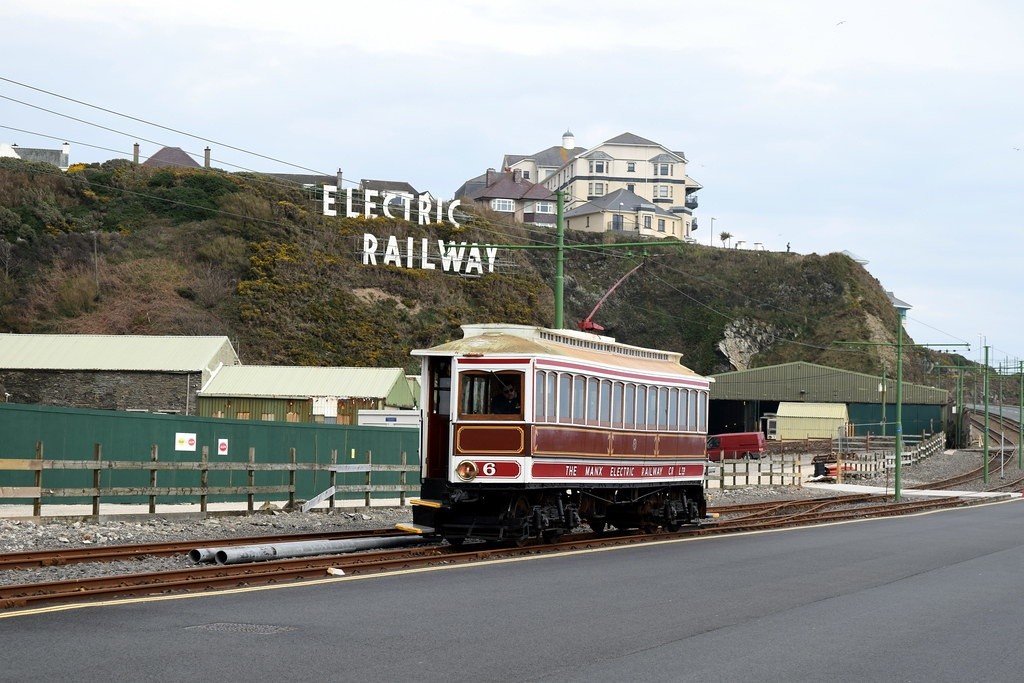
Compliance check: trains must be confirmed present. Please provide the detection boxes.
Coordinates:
[393,331,717,552]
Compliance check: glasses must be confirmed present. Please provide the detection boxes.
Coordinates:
[502,386,514,393]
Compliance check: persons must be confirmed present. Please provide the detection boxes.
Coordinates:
[502,383,521,415]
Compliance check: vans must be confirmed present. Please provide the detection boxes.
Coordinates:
[706,432,767,463]
